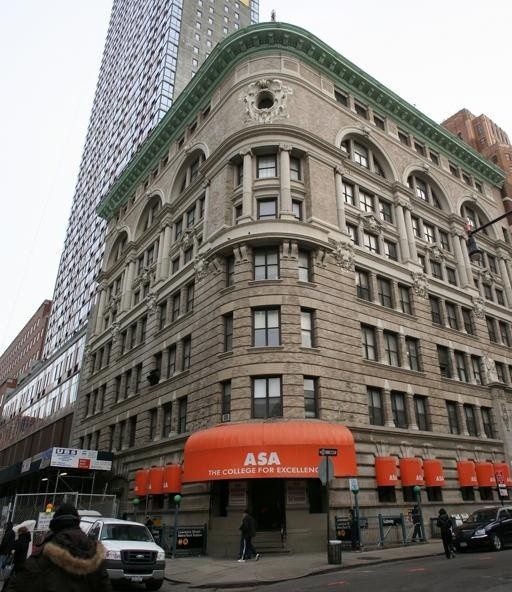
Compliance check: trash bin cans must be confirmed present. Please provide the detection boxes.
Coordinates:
[327,542,341,564]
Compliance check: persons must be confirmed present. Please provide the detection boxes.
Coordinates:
[0,521,15,570]
[347,509,359,552]
[9,527,31,574]
[436,508,456,559]
[237,507,260,563]
[412,511,424,542]
[0,503,114,592]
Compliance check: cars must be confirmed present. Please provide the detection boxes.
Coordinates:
[452,507,512,551]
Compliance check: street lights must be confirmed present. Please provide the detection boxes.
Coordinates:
[352,490,364,547]
[171,495,181,559]
[132,499,140,521]
[53,473,67,505]
[42,478,49,512]
[414,486,427,542]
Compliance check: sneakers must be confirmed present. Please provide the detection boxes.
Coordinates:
[236,552,261,562]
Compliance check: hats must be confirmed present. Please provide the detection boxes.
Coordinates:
[48,503,81,531]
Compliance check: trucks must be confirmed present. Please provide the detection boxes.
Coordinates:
[79,516,165,591]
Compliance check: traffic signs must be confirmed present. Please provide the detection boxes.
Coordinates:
[320,449,337,456]
